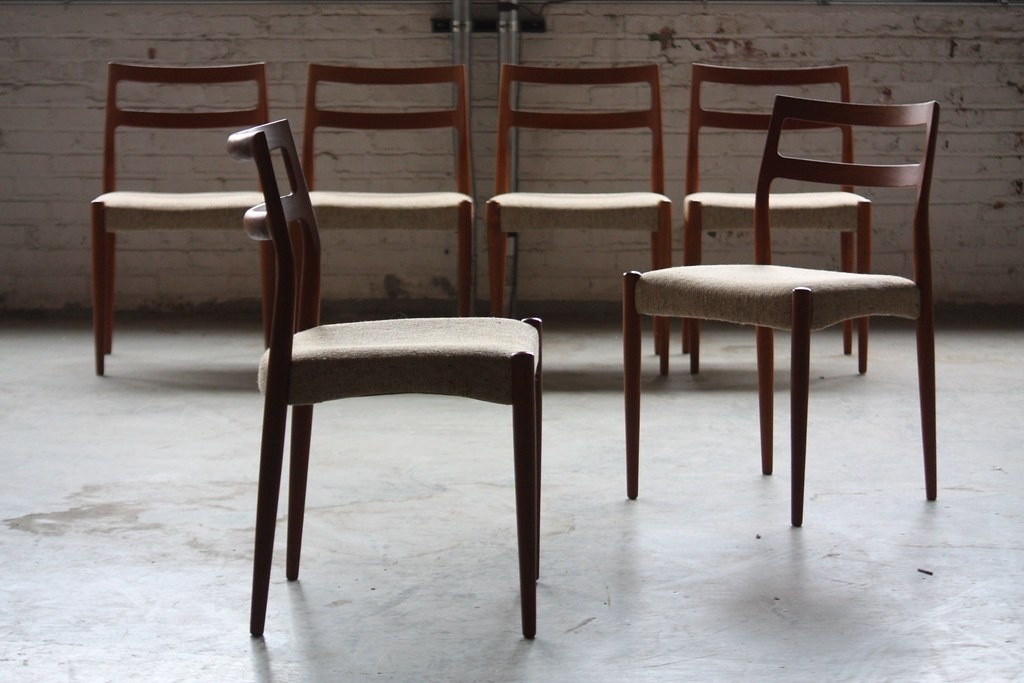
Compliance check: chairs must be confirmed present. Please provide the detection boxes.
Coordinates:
[92,62,278,377]
[294,62,475,335]
[620,93,940,528]
[486,64,675,374]
[683,63,871,378]
[227,117,544,640]
